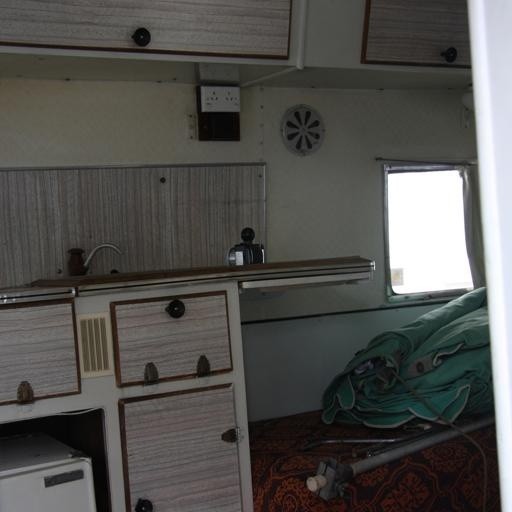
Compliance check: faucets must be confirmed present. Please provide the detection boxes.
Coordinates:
[67,240,123,277]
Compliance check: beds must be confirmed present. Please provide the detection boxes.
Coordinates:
[240,298,501,511]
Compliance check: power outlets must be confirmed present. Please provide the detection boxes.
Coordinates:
[201,86,241,112]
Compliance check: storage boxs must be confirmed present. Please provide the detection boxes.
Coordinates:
[0,440,101,512]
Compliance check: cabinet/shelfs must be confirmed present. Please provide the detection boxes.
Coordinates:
[1,1,471,89]
[0,163,377,511]
